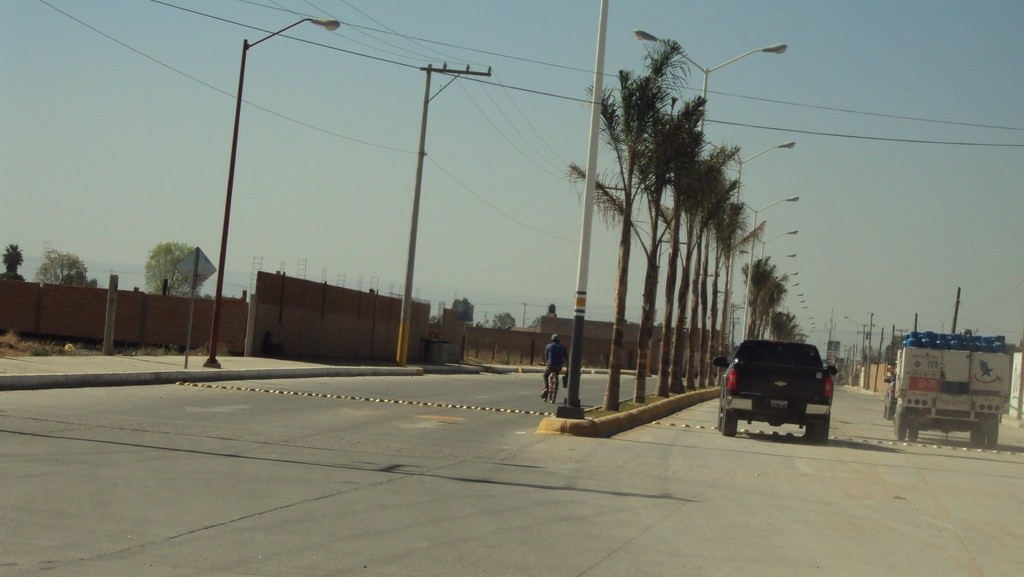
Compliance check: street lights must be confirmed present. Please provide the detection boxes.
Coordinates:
[205,15,341,370]
[630,26,835,401]
[842,313,862,387]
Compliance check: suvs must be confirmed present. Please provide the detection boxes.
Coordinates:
[710,337,839,442]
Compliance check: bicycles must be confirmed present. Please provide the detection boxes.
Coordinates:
[536,359,562,403]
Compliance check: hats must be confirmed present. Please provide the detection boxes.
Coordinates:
[551,334,560,341]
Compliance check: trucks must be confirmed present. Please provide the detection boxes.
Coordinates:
[892,326,1015,450]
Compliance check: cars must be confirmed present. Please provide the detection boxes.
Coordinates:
[880,376,901,419]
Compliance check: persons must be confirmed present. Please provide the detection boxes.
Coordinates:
[887,365,896,375]
[541,334,569,399]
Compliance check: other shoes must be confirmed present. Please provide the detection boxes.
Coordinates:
[541,388,548,399]
